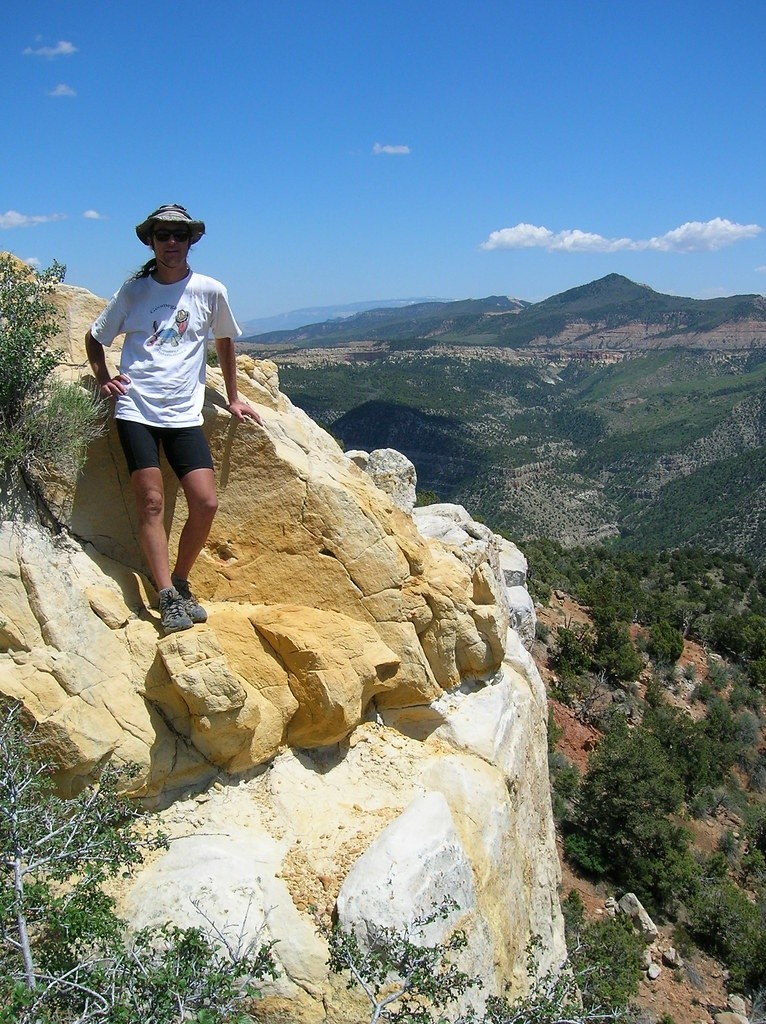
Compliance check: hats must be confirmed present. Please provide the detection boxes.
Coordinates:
[135,204,205,246]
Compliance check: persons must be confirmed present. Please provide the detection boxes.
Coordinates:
[85,204,263,636]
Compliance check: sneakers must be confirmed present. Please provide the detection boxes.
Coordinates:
[159,590,194,634]
[177,586,207,623]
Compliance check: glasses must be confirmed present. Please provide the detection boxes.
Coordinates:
[152,229,191,242]
